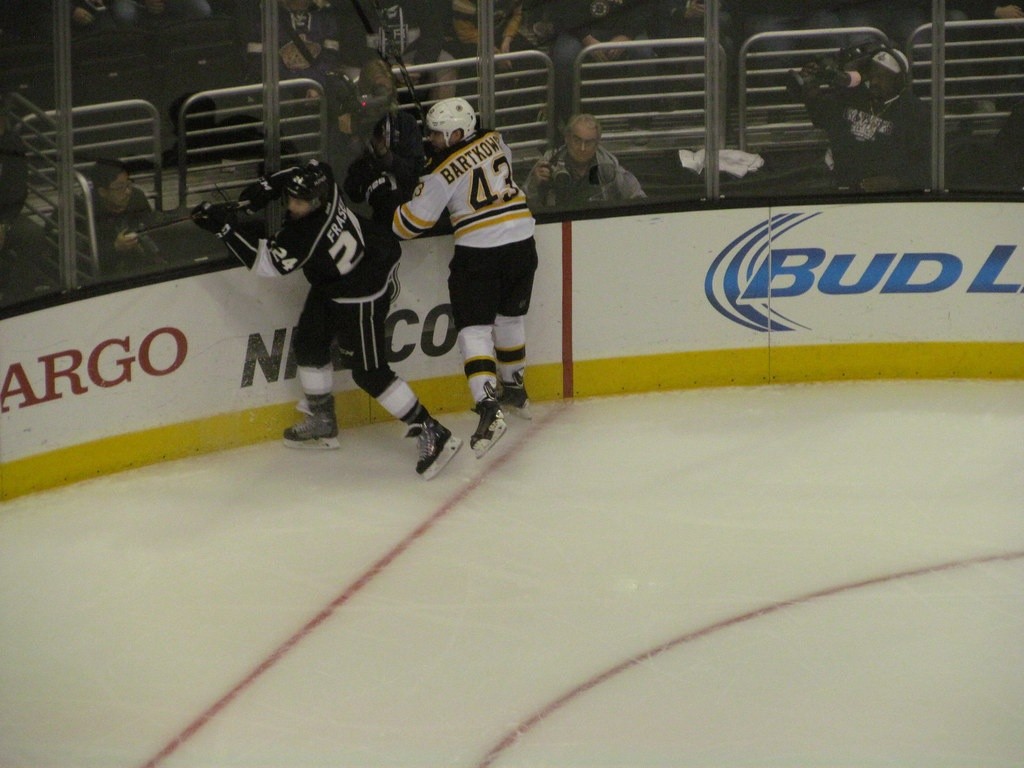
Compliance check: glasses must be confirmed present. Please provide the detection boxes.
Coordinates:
[102,179,135,193]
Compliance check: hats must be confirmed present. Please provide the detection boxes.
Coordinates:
[871,49,909,74]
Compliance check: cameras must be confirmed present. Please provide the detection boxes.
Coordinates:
[124,224,160,260]
[547,161,581,198]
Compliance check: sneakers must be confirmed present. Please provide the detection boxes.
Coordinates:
[470,381,508,459]
[283,396,340,450]
[494,367,533,420]
[405,404,463,482]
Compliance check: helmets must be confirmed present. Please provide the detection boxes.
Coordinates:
[426,97,476,148]
[287,165,328,200]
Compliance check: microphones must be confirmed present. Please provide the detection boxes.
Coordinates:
[880,88,907,107]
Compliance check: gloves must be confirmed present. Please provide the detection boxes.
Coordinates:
[192,201,236,242]
[344,169,398,204]
[239,175,280,217]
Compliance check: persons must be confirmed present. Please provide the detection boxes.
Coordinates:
[0,108,44,306]
[987,97,1023,193]
[795,43,930,189]
[979,0,1024,86]
[246,0,539,145]
[523,113,648,212]
[191,161,463,480]
[343,112,450,237]
[78,158,162,275]
[73,0,213,30]
[545,0,738,124]
[389,96,538,454]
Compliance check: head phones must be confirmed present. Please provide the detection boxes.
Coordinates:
[881,47,912,94]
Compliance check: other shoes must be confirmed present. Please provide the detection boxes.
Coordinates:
[630,126,650,145]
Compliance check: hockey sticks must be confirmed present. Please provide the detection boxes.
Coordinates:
[372,0,430,143]
[115,199,253,251]
[353,0,396,79]
